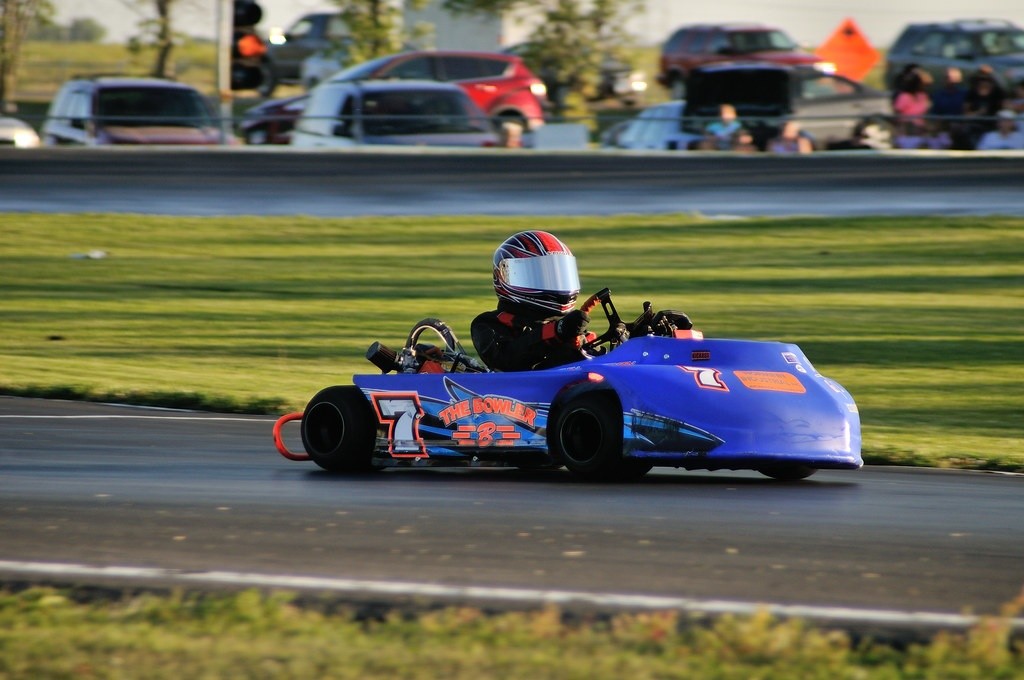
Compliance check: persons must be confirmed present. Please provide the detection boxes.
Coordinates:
[470,231,673,372]
[692,66,1024,150]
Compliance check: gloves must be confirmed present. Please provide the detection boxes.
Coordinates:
[554,310,590,341]
[651,310,692,337]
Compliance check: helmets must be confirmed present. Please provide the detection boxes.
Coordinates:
[493,231,581,317]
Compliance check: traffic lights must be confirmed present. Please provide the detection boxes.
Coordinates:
[230,2,267,92]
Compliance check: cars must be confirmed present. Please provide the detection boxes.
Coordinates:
[38,73,245,147]
[261,12,414,101]
[600,95,816,155]
[676,64,898,154]
[239,46,548,147]
[0,114,39,148]
[291,73,507,155]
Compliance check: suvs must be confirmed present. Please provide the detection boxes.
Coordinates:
[656,22,833,105]
[883,15,1024,120]
